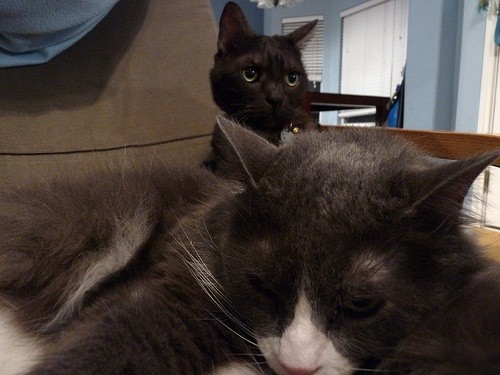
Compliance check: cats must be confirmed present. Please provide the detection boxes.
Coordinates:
[0,114,500,375]
[196,0,321,175]
